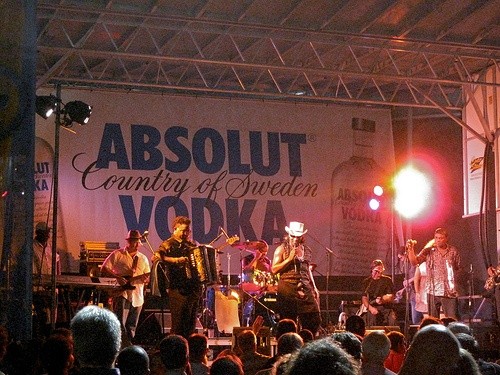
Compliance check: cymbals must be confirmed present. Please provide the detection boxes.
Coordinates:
[231,240,263,248]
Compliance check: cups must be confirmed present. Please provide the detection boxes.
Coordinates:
[208,326,214,338]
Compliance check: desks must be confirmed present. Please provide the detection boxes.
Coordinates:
[31,273,122,330]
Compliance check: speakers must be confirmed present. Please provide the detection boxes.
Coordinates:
[135,313,203,345]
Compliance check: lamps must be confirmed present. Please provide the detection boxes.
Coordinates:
[64,101,93,126]
[36,93,59,120]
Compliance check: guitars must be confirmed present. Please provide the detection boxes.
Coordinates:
[118,272,152,292]
[356,299,376,317]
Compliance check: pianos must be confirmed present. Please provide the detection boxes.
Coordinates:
[32,273,120,297]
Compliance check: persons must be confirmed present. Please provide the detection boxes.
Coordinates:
[101,230,151,338]
[398,228,462,320]
[0,306,273,375]
[360,259,396,326]
[483,265,500,323]
[240,239,276,327]
[151,216,219,339]
[20,222,61,325]
[271,221,321,336]
[255,316,500,375]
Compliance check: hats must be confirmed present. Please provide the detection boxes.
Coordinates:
[36,222,53,231]
[285,222,308,237]
[125,229,142,240]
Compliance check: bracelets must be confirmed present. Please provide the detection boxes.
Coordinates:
[175,258,180,264]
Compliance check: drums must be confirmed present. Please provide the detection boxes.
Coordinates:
[238,269,266,293]
[262,271,280,293]
[242,293,278,332]
[207,284,240,315]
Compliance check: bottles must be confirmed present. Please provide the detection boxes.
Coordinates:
[328,117,392,306]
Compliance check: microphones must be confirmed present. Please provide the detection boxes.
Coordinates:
[142,231,148,238]
[293,238,297,272]
[408,240,417,244]
